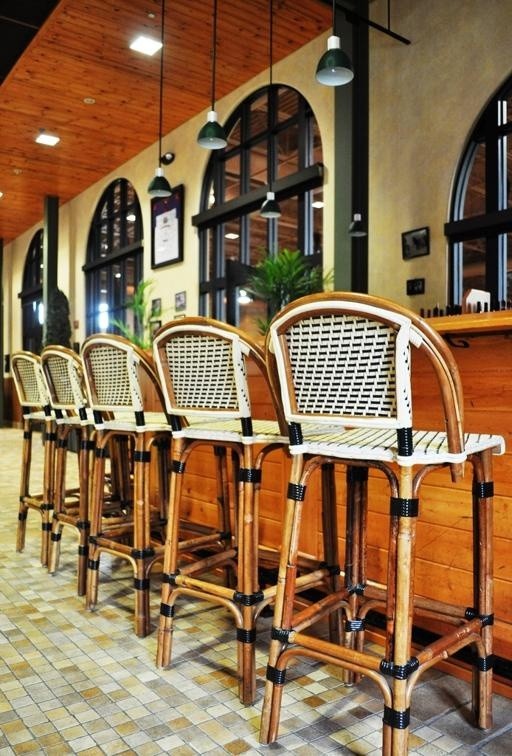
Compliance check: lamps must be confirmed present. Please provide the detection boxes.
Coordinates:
[314,0,355,87]
[260,0,282,217]
[198,0,228,149]
[147,0,172,197]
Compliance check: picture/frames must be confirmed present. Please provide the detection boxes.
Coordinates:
[149,184,184,269]
[406,279,425,295]
[402,226,430,260]
